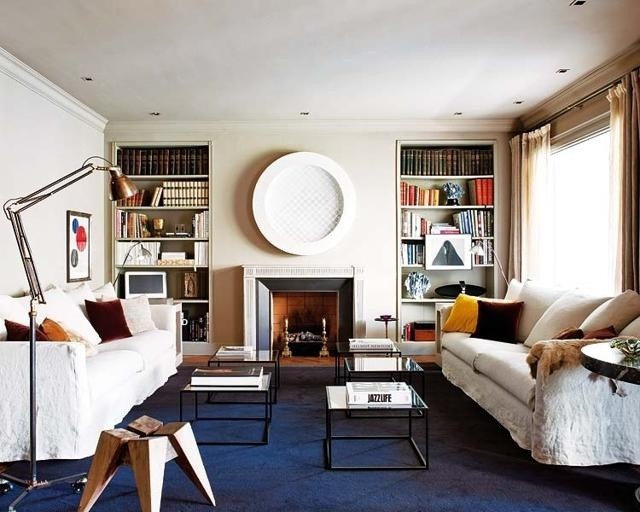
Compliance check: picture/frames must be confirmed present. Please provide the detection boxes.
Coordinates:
[64,208,93,284]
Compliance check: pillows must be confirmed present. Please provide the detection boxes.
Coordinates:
[82,297,135,341]
[502,279,614,349]
[440,289,514,334]
[1,281,121,326]
[468,293,527,343]
[39,317,71,348]
[580,287,639,340]
[100,294,157,333]
[52,317,96,355]
[34,288,103,346]
[3,317,49,348]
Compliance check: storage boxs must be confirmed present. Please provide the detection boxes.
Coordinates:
[412,321,436,342]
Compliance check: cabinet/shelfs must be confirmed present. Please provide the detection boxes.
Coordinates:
[396,138,502,347]
[110,138,212,358]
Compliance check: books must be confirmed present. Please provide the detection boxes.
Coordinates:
[191,365,264,387]
[115,146,210,343]
[346,382,411,406]
[348,338,394,350]
[401,148,494,341]
[215,345,252,356]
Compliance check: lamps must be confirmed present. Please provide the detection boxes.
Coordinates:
[1,151,143,511]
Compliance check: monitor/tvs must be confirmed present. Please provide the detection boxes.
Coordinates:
[124,271,167,298]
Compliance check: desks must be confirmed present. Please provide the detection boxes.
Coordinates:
[574,337,640,504]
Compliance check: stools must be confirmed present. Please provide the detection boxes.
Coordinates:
[85,413,220,512]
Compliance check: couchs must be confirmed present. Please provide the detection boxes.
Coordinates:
[432,278,639,477]
[1,293,186,465]
